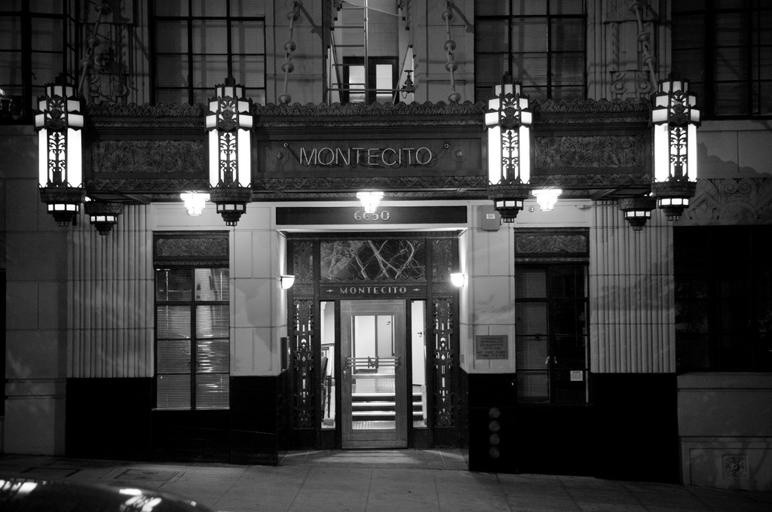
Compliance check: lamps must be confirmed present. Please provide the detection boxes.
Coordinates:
[355,190,385,216]
[202,1,257,229]
[530,189,563,212]
[651,1,703,220]
[492,198,524,224]
[84,203,122,235]
[179,193,211,217]
[617,197,657,231]
[480,1,535,223]
[280,274,295,290]
[450,272,464,287]
[31,1,86,229]
